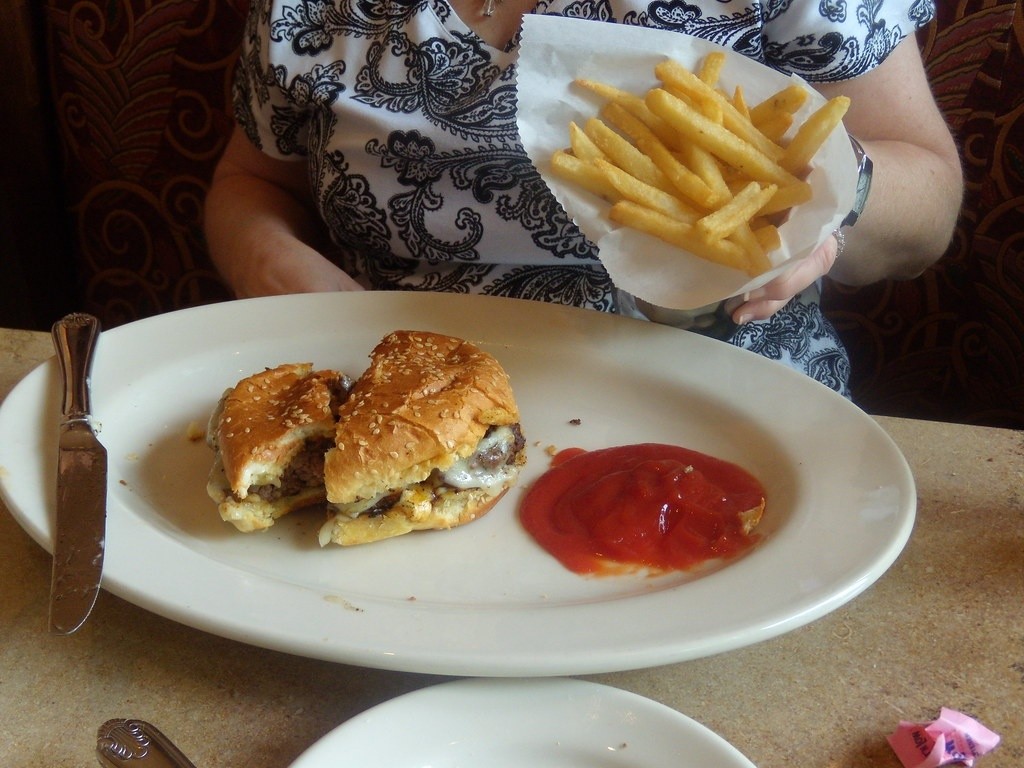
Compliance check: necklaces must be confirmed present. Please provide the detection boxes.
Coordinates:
[480,0,503,16]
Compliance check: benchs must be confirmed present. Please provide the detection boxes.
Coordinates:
[0,0,1023,430]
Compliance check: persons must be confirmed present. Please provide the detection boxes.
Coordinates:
[206,0,963,403]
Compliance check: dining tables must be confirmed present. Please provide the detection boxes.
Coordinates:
[0,325,1024,765]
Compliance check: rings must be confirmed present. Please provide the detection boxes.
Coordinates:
[832,229,845,257]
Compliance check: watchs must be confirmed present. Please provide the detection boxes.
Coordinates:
[833,136,873,225]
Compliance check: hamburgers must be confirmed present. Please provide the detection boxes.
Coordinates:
[204,328,525,547]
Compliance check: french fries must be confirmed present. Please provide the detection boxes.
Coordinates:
[550,50,851,278]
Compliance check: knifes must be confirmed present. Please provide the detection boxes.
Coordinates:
[45,312,107,636]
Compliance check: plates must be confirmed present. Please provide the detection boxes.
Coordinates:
[0,292,916,676]
[292,674,761,768]
